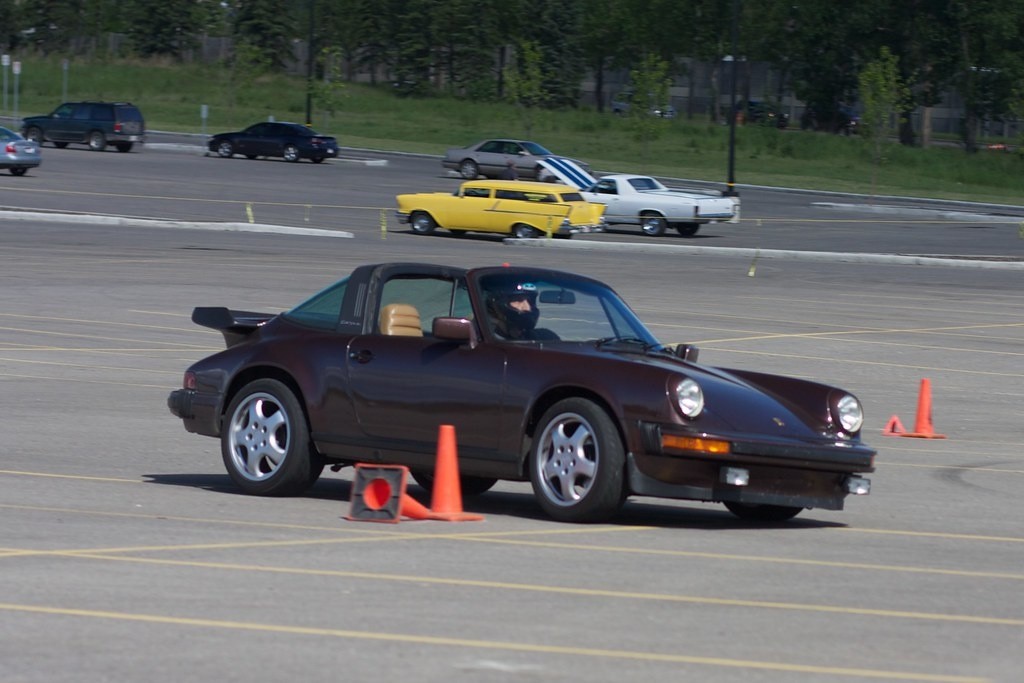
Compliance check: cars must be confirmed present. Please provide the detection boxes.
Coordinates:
[611,90,678,120]
[394,180,608,240]
[801,104,867,137]
[0,127,42,177]
[535,156,742,238]
[206,120,340,163]
[439,137,593,185]
[724,101,790,129]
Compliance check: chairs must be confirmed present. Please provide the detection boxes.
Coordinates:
[379,303,424,336]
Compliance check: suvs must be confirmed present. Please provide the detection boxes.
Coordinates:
[18,101,148,152]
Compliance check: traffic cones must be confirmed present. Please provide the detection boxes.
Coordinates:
[898,378,946,439]
[350,463,432,524]
[424,426,487,524]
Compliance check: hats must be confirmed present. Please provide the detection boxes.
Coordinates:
[507,159,517,164]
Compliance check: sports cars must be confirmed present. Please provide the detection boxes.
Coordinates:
[163,260,882,528]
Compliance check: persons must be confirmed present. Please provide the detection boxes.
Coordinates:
[501,161,519,180]
[484,279,561,341]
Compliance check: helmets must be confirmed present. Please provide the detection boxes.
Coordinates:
[485,281,540,330]
